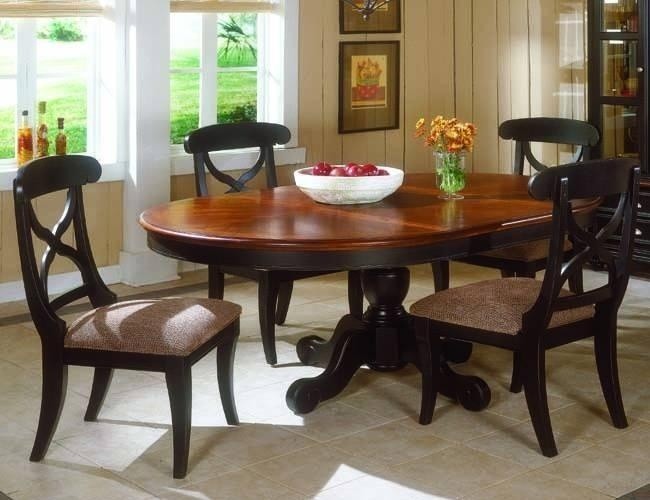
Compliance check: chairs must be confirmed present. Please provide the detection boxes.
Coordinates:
[183,121,365,366]
[432,117,601,294]
[12,154,241,479]
[409,157,641,457]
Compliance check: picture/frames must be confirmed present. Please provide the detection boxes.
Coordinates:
[338,0,401,35]
[337,41,400,134]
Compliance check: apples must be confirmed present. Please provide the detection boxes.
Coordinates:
[348,165,362,177]
[358,163,379,176]
[313,161,330,176]
[345,162,357,175]
[379,170,389,175]
[328,167,346,176]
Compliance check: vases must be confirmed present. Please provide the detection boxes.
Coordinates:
[433,150,467,201]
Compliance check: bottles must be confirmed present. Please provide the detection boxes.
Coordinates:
[18,110,32,167]
[37,102,50,158]
[56,118,67,156]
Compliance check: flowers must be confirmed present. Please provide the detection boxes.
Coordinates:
[415,114,477,191]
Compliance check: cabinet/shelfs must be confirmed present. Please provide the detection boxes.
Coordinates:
[587,0,650,280]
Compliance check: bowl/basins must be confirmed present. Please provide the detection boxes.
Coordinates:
[296,165,405,205]
